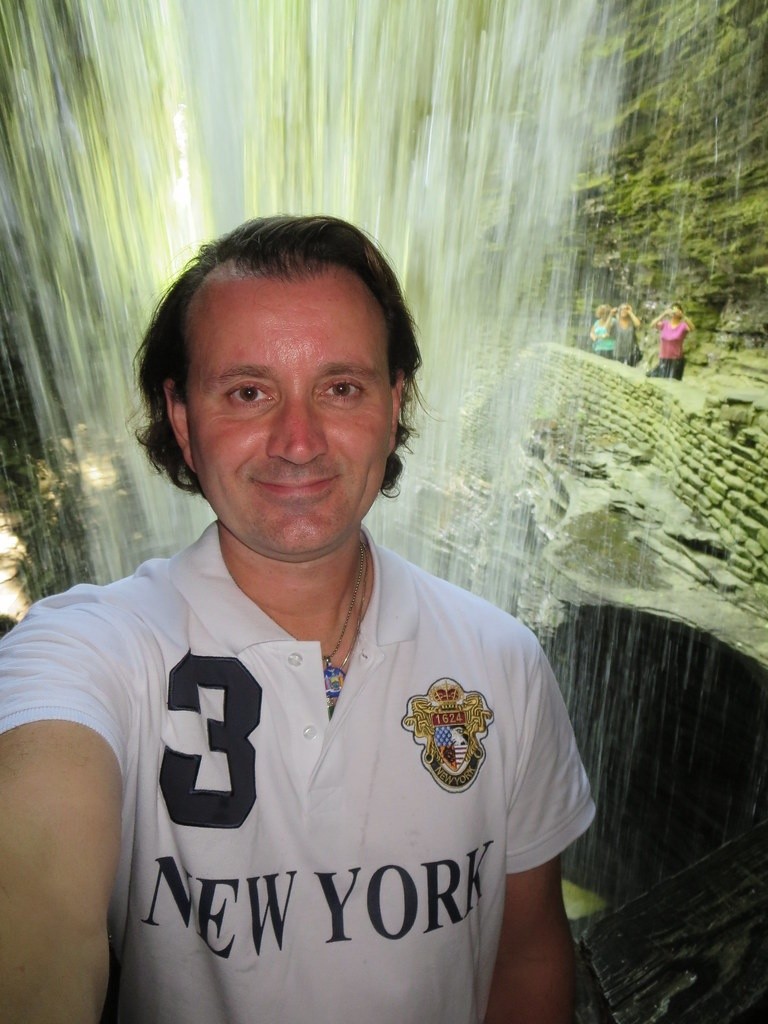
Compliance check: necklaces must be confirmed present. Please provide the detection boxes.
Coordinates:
[323,540,368,717]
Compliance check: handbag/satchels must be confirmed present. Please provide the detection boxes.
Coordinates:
[626,342,643,368]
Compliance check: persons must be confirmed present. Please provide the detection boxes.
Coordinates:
[605,304,640,367]
[590,305,615,360]
[651,303,695,379]
[0,216,596,1024]
